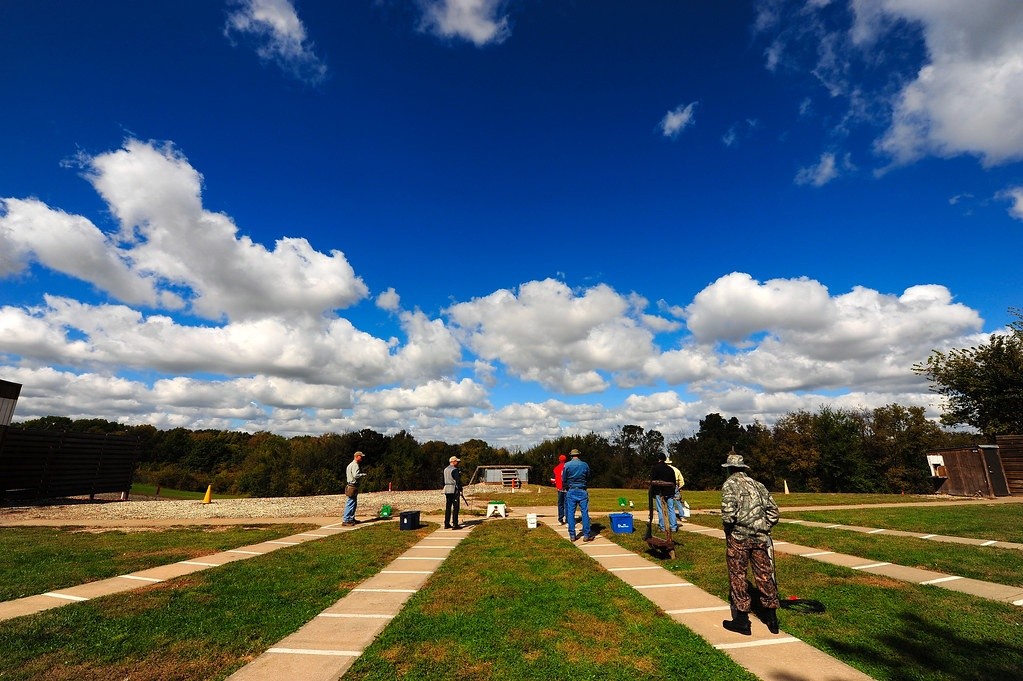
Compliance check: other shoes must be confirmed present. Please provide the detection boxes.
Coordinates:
[571,536,576,541]
[661,528,665,532]
[352,519,360,524]
[445,523,451,528]
[671,527,676,532]
[342,521,354,526]
[583,536,595,541]
[558,521,563,525]
[566,523,578,527]
[681,518,687,521]
[452,523,460,530]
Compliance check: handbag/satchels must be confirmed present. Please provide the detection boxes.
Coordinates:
[345,485,358,496]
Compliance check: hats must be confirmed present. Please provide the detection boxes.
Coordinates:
[722,454,750,468]
[449,456,461,462]
[569,449,581,455]
[664,457,672,463]
[354,451,365,456]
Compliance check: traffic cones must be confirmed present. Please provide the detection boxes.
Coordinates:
[200,482,212,504]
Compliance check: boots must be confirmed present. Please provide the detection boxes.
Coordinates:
[723,610,752,635]
[760,608,778,634]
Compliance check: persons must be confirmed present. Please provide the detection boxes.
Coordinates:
[441,456,463,530]
[721,455,780,635]
[553,449,591,542]
[649,452,687,533]
[342,451,367,527]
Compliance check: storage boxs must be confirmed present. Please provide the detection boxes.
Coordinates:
[399,511,420,531]
[526,514,537,529]
[609,513,633,534]
